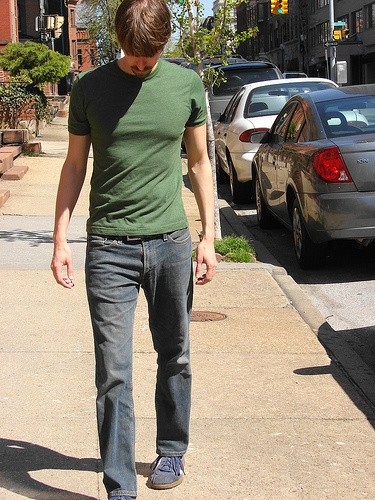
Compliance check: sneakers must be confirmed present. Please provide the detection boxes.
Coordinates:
[107,495,136,500]
[148,454,186,490]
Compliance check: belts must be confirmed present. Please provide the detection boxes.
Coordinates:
[90,229,174,245]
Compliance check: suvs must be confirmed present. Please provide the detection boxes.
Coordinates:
[161,55,310,154]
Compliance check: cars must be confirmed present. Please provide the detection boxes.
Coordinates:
[214,78,369,201]
[251,84,375,269]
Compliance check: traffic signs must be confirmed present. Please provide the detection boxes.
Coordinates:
[323,41,338,48]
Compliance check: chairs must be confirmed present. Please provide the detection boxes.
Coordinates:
[249,102,268,113]
[220,74,245,90]
[324,111,364,134]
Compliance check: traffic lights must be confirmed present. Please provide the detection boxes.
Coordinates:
[334,24,349,40]
[271,0,289,14]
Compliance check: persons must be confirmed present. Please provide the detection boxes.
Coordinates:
[50,1,217,500]
